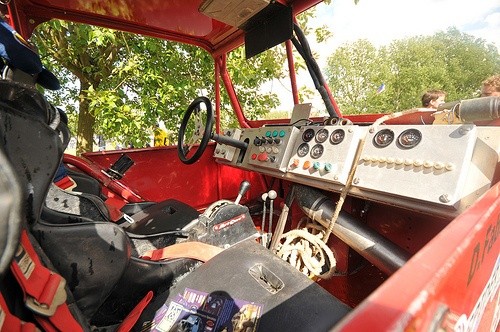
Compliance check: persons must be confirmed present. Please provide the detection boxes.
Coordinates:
[421,89,446,108]
[481,75,500,97]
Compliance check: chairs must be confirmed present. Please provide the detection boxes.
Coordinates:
[6,80,201,261]
[0,105,354,332]
